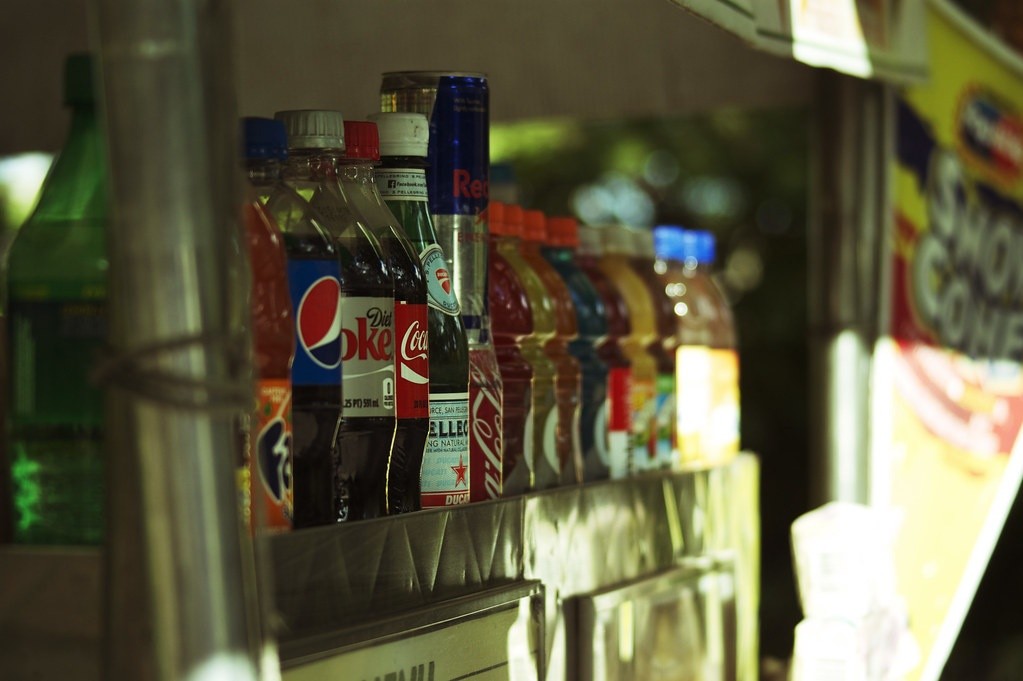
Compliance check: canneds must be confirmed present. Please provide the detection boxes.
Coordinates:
[379,69,506,503]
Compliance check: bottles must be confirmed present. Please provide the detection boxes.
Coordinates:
[0,52,109,547]
[240,109,742,535]
